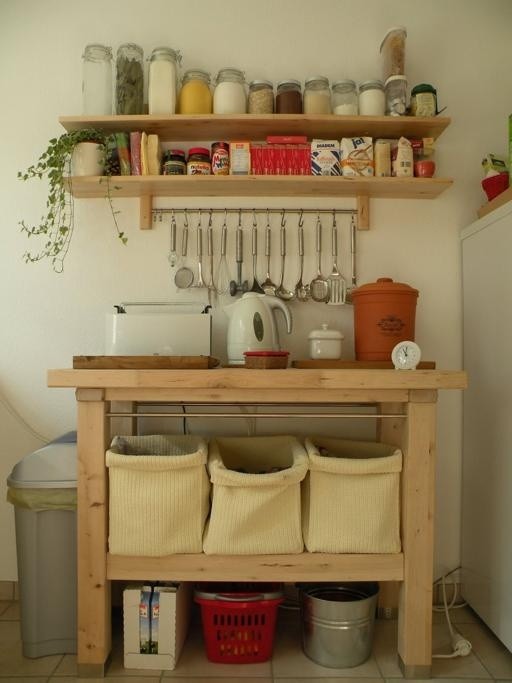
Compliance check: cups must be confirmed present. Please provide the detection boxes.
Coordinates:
[413,158,437,179]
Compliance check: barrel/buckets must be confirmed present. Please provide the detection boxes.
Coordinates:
[296,582,381,667]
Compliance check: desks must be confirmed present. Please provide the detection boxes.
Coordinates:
[45,364,472,681]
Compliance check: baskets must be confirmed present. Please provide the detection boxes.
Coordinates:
[192,585,286,664]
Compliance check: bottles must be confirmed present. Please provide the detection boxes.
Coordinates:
[303,76,333,114]
[274,80,302,113]
[178,69,213,114]
[357,79,386,115]
[331,78,358,115]
[114,43,145,115]
[247,80,275,113]
[82,42,114,116]
[147,46,177,114]
[213,70,247,114]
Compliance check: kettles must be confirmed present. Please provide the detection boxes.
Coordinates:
[221,290,292,364]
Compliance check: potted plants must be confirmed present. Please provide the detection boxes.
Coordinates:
[11,123,130,275]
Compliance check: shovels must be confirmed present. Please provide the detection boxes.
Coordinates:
[325,219,346,306]
[261,227,277,296]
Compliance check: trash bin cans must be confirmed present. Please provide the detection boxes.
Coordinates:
[7,431,77,658]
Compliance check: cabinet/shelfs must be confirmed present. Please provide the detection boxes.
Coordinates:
[55,109,455,230]
